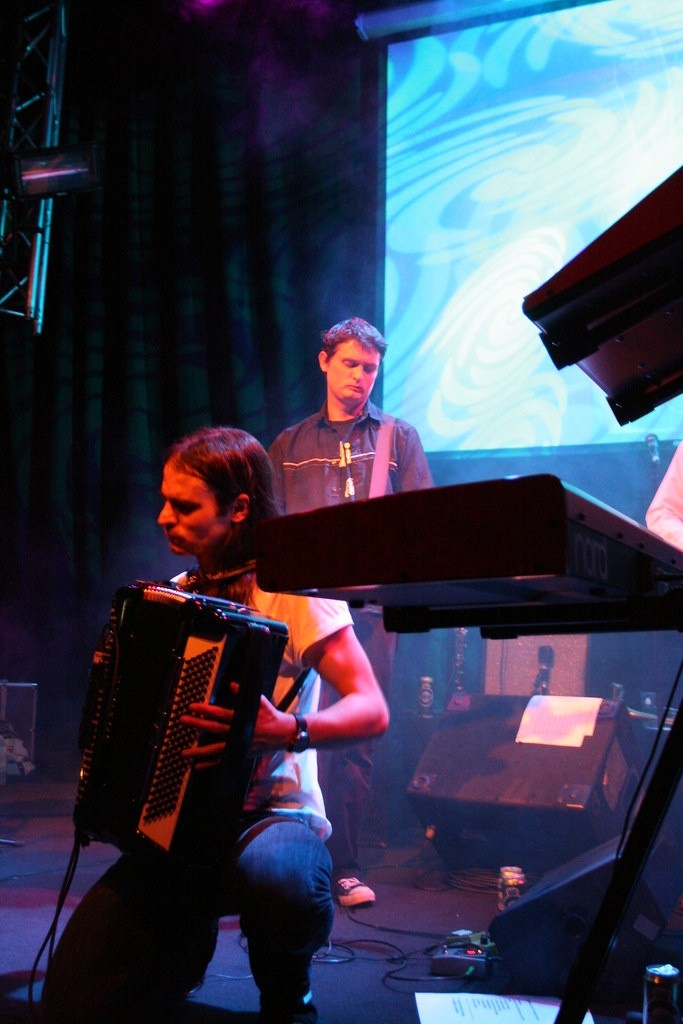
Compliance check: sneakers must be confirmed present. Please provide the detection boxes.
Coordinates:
[337,877,375,906]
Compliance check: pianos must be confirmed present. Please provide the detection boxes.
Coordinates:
[60,570,288,856]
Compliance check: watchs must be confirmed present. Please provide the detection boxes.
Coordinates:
[288,710,311,755]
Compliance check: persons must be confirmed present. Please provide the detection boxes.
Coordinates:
[45,428,391,1024]
[269,319,432,911]
[647,443,682,555]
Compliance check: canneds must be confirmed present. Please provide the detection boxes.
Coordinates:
[497,866,527,910]
[419,676,434,719]
[642,963,680,1024]
